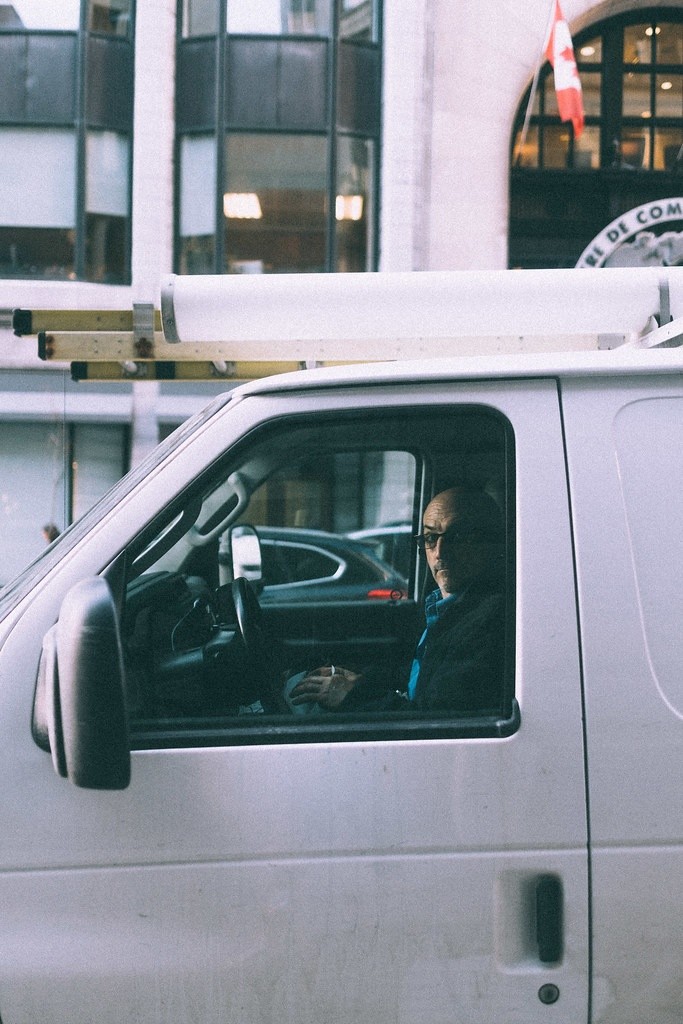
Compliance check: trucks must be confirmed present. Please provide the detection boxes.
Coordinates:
[0,265,683,1024]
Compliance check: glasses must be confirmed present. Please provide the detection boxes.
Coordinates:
[412,532,477,550]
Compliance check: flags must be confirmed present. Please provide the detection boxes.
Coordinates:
[545,0,584,138]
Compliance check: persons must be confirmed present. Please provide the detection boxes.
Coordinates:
[291,486,512,714]
[43,525,59,543]
